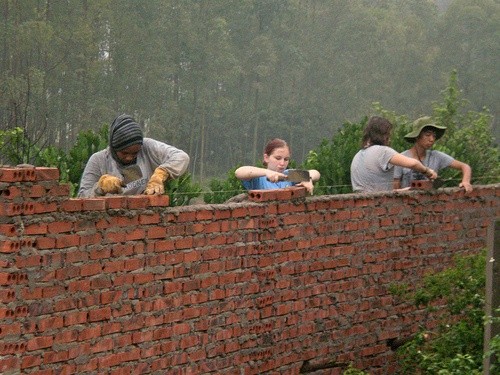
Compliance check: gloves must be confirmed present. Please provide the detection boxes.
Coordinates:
[98,174,124,194]
[144,168,169,195]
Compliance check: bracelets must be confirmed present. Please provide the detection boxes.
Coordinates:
[422,167,428,174]
[309,176,314,183]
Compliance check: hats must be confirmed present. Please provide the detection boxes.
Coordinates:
[403,117,448,143]
[111,113,142,151]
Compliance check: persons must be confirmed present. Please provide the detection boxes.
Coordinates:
[234,138,321,197]
[350,116,437,194]
[78,114,190,198]
[394,117,473,198]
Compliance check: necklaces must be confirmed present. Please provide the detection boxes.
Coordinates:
[414,146,425,164]
